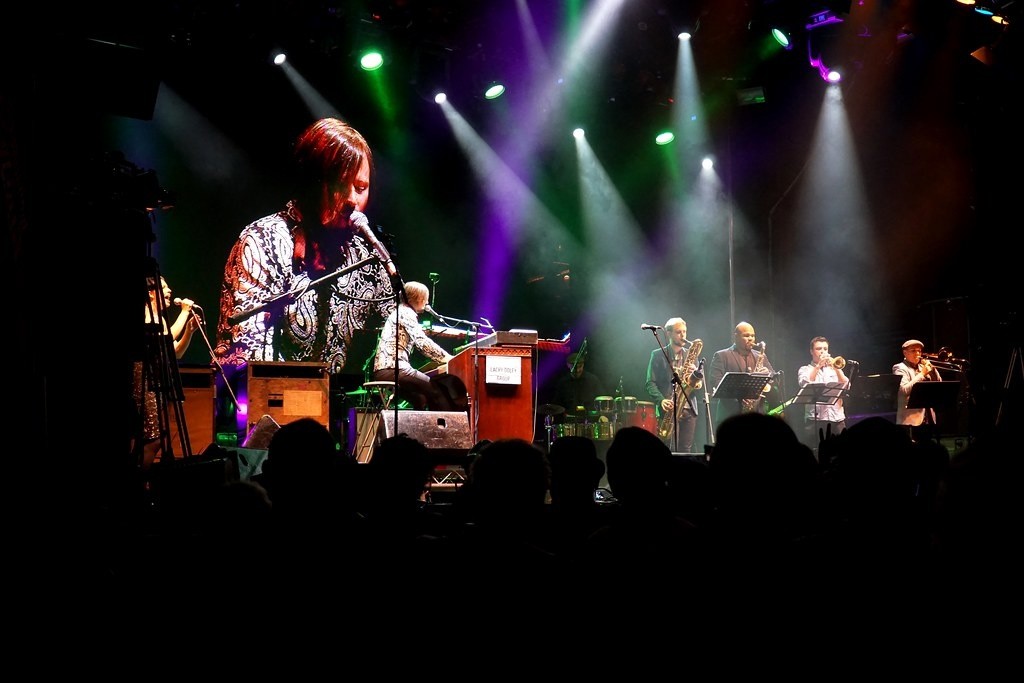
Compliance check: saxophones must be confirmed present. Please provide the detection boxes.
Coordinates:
[656,338,704,439]
[742,341,771,410]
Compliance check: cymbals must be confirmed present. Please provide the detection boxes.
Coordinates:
[539,404,565,414]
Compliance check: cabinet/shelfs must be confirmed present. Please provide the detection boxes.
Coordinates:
[128,367,215,456]
[245,360,331,435]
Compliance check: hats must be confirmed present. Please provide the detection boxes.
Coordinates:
[567,353,584,365]
[902,339,924,349]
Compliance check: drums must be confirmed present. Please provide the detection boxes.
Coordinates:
[559,423,587,437]
[594,396,614,410]
[614,396,637,413]
[587,422,614,441]
[615,401,656,436]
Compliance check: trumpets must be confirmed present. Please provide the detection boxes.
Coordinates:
[827,356,846,369]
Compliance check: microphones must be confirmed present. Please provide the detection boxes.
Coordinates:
[847,360,859,365]
[952,358,967,362]
[698,359,704,371]
[349,212,398,277]
[584,339,588,355]
[757,342,765,348]
[174,298,201,310]
[640,323,661,330]
[424,305,446,325]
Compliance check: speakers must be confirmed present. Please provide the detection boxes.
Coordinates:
[597,450,709,506]
[241,414,281,450]
[373,410,476,468]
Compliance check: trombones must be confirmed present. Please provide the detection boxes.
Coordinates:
[917,347,970,373]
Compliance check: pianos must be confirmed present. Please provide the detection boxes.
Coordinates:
[418,327,539,441]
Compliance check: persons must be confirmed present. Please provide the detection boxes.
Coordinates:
[707,321,780,426]
[555,353,606,415]
[797,337,851,461]
[216,117,399,394]
[0,412,1024,683]
[373,281,455,410]
[646,316,701,454]
[130,276,204,494]
[892,340,943,451]
